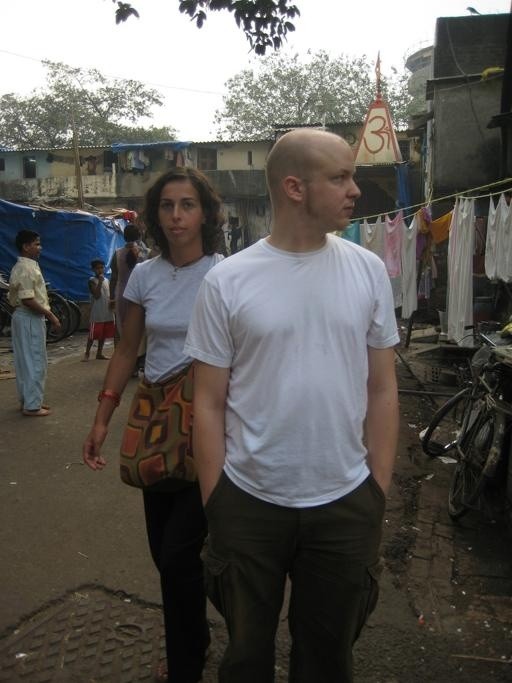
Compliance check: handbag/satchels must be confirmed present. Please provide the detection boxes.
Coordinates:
[120,365,197,488]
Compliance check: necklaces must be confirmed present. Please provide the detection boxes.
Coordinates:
[167,253,205,280]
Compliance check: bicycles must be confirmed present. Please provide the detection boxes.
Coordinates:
[1,272,81,343]
[422,325,511,519]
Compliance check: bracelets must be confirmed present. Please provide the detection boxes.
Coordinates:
[97,390,121,407]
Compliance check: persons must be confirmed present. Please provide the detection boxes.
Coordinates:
[181,129,401,683]
[7,229,61,417]
[83,163,228,683]
[109,225,148,378]
[81,260,116,362]
